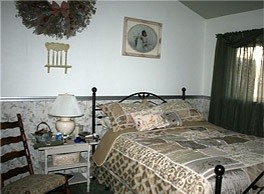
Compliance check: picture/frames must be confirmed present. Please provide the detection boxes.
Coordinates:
[122,17,162,59]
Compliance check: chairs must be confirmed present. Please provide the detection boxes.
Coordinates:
[0,113,69,194]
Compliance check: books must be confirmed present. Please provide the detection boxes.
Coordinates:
[78,132,94,143]
[54,173,74,181]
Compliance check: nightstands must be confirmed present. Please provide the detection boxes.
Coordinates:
[37,139,101,192]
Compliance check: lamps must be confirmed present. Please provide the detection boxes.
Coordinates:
[48,94,84,139]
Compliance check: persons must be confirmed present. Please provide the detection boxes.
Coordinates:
[139,30,149,52]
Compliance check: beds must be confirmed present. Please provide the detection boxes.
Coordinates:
[90,87,264,194]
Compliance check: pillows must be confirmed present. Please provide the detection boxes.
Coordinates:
[128,102,183,133]
[163,100,205,124]
[97,101,158,133]
